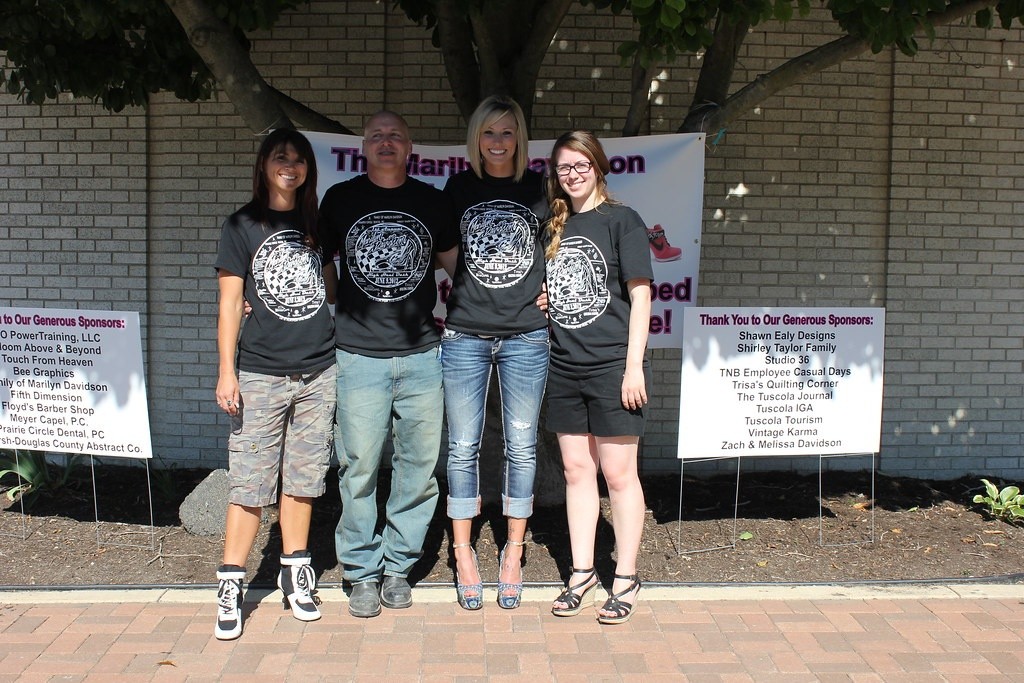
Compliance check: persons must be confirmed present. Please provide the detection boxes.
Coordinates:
[241,109,550,618]
[542,130,654,625]
[213,125,339,642]
[442,93,551,611]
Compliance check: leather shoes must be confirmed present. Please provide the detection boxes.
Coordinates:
[349,583,381,617]
[381,575,412,608]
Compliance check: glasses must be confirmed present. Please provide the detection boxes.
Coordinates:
[553,161,591,176]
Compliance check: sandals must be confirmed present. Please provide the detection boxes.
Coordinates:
[498,540,525,608]
[453,544,483,609]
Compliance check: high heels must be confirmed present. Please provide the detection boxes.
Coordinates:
[552,567,599,616]
[598,574,641,624]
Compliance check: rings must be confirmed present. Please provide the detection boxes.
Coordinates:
[216,400,221,405]
[227,399,232,405]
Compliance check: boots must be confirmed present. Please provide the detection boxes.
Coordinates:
[214,564,246,640]
[277,550,321,622]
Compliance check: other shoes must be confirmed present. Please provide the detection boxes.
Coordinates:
[646,224,681,262]
